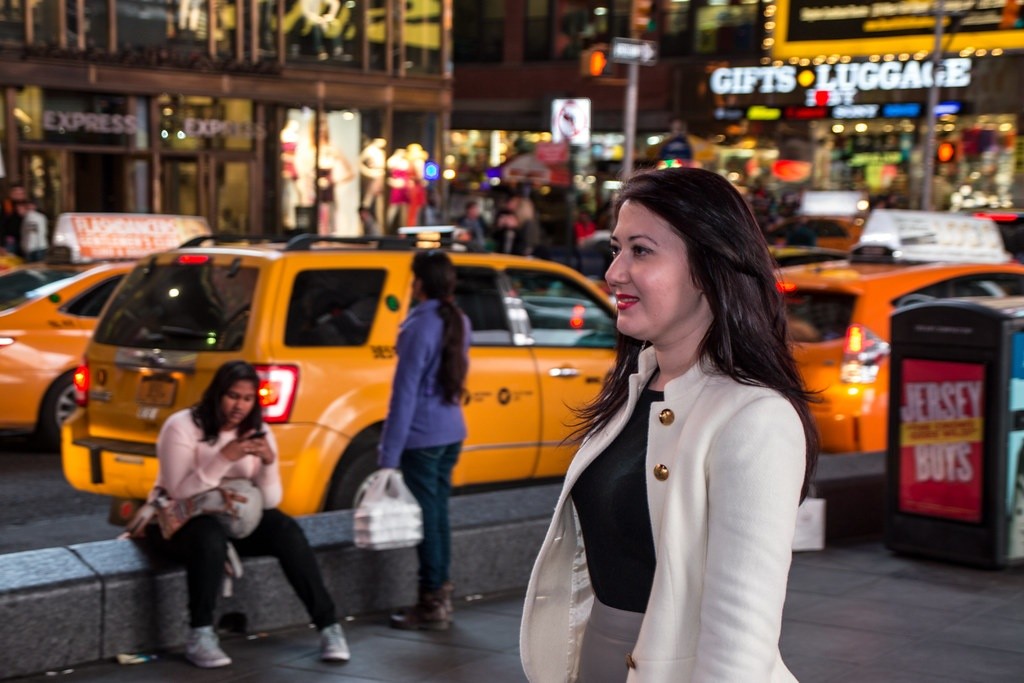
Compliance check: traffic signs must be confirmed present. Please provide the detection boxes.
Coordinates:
[611,36,659,65]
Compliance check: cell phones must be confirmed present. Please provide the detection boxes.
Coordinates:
[248,431,266,439]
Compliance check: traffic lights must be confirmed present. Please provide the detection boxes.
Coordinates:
[937,142,959,165]
[584,48,619,79]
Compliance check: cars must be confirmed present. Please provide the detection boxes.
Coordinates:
[762,215,861,254]
[0,260,137,453]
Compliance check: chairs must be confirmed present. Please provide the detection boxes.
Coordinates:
[456,273,529,331]
[313,311,368,346]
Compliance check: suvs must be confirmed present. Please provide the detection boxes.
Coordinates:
[59,226,622,516]
[772,243,1024,456]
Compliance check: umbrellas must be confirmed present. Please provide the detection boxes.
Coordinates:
[493,152,571,190]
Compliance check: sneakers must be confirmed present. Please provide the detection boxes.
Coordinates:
[184,626,231,667]
[320,622,351,660]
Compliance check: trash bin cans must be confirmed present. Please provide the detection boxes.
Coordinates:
[878,296,1023,570]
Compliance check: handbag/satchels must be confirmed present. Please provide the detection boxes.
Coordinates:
[216,479,264,535]
[353,467,423,551]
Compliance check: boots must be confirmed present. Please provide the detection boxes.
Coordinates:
[389,590,448,630]
[441,581,454,625]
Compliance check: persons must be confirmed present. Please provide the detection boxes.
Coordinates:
[0,183,47,256]
[376,248,471,632]
[127,360,352,669]
[462,195,541,264]
[518,166,821,683]
[276,116,427,238]
